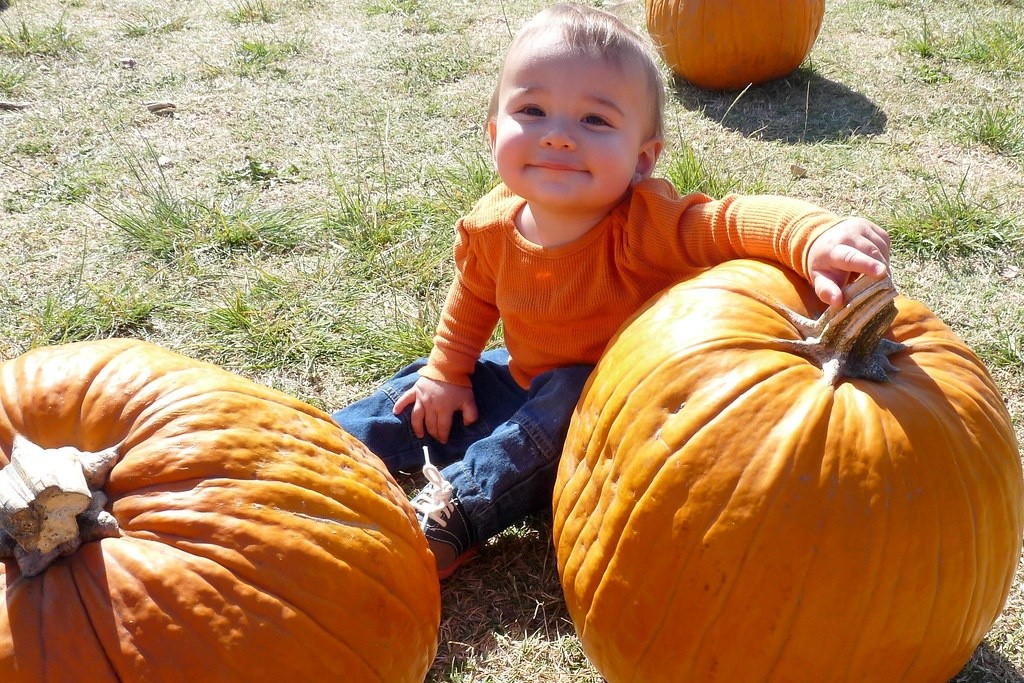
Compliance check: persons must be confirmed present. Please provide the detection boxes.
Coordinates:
[332,3,889,578]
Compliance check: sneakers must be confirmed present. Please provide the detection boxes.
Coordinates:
[402,445,482,579]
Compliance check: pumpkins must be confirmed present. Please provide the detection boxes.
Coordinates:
[551,259,1024,683]
[0,337,442,683]
[644,0,826,90]
